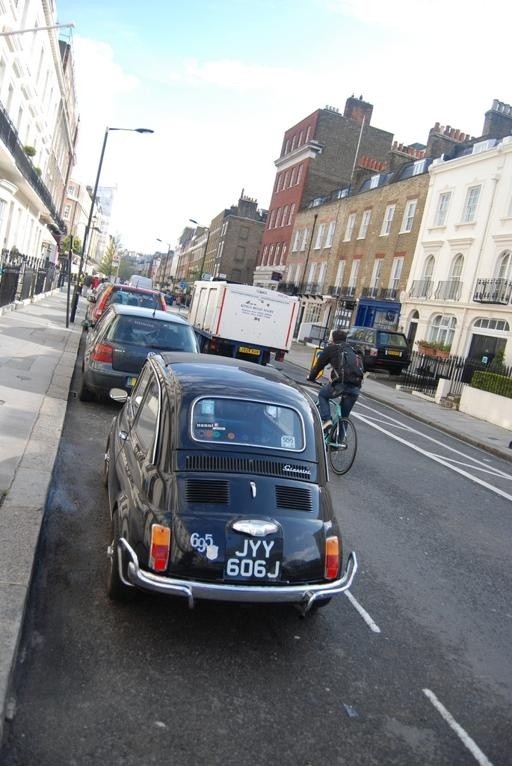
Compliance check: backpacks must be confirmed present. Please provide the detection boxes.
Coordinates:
[329,341,366,387]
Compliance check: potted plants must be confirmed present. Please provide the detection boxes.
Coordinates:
[415,337,453,358]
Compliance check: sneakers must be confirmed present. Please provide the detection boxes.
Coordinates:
[321,419,334,431]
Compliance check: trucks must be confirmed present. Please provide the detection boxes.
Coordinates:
[188,281,301,366]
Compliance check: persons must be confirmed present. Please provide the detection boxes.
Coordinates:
[79,272,101,297]
[308,330,363,452]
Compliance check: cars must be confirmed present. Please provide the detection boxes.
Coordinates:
[124,275,152,290]
[346,326,410,375]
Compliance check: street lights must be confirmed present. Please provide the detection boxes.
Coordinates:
[189,219,210,280]
[156,238,171,288]
[70,126,155,323]
